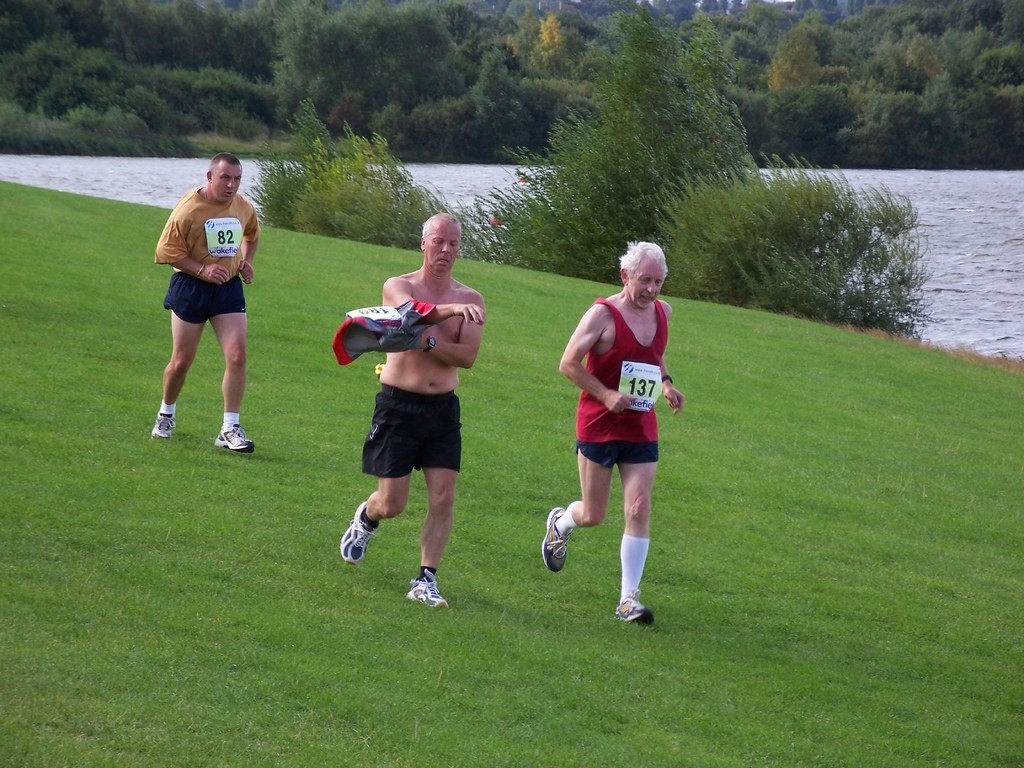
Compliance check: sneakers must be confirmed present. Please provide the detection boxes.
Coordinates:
[152,412,176,438]
[214,424,254,453]
[615,590,654,624]
[340,501,378,564]
[542,507,573,572]
[406,568,449,607]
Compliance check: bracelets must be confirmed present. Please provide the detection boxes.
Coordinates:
[194,263,207,277]
[661,374,673,383]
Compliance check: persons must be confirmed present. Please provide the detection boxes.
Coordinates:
[541,238,682,626]
[150,153,260,451]
[337,209,486,611]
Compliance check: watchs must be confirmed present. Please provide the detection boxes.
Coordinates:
[422,335,437,353]
